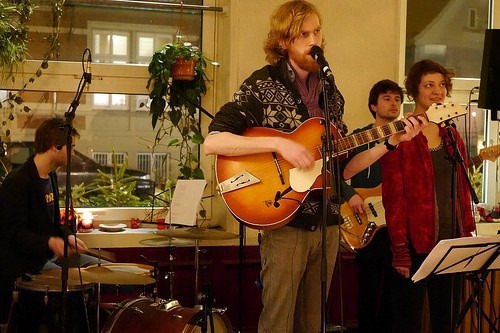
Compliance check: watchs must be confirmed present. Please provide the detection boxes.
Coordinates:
[385,139,398,151]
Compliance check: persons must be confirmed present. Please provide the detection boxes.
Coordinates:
[0,118,113,276]
[379,59,476,333]
[339,80,404,333]
[203,0,428,333]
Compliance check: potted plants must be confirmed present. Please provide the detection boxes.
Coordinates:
[146,36,221,181]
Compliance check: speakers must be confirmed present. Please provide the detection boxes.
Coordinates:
[477,29,500,111]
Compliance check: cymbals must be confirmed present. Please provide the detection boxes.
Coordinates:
[152,228,239,240]
[39,265,157,286]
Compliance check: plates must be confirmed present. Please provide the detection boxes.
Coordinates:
[98,224,127,232]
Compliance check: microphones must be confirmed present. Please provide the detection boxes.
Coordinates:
[309,45,335,86]
[85,54,92,91]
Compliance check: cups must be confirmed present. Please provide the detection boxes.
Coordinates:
[80,217,94,229]
[131,218,139,229]
[156,218,166,229]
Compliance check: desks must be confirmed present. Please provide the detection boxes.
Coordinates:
[77,227,241,248]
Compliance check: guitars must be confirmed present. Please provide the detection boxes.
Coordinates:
[215,103,467,230]
[338,138,500,250]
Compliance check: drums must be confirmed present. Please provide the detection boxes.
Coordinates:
[99,296,228,333]
[13,273,100,333]
[87,262,160,304]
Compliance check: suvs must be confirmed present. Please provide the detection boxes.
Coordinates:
[0,141,157,202]
